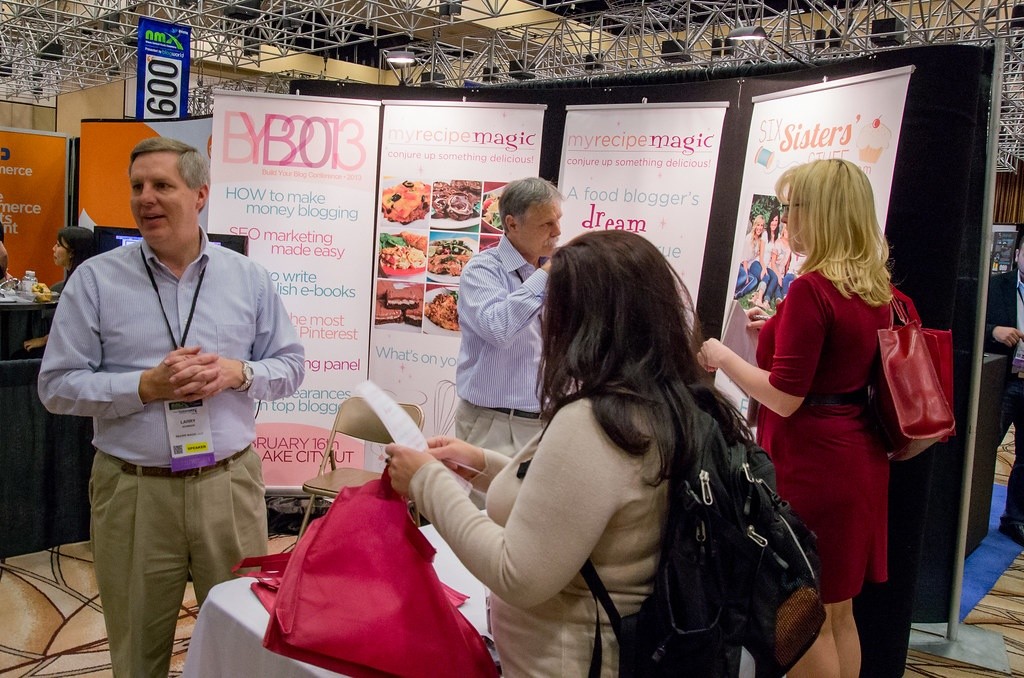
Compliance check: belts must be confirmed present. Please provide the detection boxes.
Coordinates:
[488,406,552,421]
[100,444,251,478]
[803,390,867,405]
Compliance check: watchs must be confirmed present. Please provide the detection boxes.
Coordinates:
[233,361,254,391]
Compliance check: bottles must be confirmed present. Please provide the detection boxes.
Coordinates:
[21,270,38,303]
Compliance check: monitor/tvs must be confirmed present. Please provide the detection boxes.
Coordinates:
[91,225,249,257]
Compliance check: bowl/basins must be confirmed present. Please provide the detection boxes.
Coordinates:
[482,182,511,235]
[15,291,59,304]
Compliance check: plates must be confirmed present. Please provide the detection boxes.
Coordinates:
[429,212,480,231]
[422,286,463,338]
[426,239,477,285]
[381,232,428,276]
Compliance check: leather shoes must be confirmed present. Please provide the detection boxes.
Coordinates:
[999,521,1024,548]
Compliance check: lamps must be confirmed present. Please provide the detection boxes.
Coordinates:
[244,38,259,57]
[585,12,604,72]
[814,0,842,51]
[711,0,734,59]
[387,51,415,64]
[439,0,462,18]
[102,12,120,32]
[726,26,767,40]
[482,67,499,83]
[1009,0,1024,29]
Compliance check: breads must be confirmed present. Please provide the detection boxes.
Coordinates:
[32,283,51,302]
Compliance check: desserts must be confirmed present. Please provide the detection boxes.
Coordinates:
[431,181,483,220]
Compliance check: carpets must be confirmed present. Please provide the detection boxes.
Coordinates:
[961,483,1024,620]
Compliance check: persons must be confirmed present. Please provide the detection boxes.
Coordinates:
[23,225,94,351]
[38,136,305,678]
[696,158,892,678]
[748,209,780,311]
[385,229,716,678]
[983,226,1024,546]
[782,252,807,300]
[771,223,791,305]
[734,215,765,300]
[454,177,567,493]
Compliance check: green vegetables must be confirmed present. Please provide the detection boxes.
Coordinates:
[435,239,468,263]
[490,212,503,230]
[379,232,408,248]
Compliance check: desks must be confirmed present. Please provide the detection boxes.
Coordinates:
[1,299,60,360]
[181,509,504,678]
[966,353,1010,556]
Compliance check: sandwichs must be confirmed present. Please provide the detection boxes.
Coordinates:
[375,281,424,327]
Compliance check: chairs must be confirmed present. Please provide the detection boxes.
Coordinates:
[297,397,424,539]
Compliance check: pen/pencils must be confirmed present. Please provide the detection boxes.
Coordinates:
[752,315,773,323]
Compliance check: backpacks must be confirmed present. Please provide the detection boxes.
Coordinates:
[529,382,828,678]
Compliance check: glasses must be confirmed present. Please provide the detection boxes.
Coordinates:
[778,202,808,215]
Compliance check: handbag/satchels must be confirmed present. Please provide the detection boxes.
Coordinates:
[891,282,955,442]
[868,288,956,463]
[230,475,501,678]
